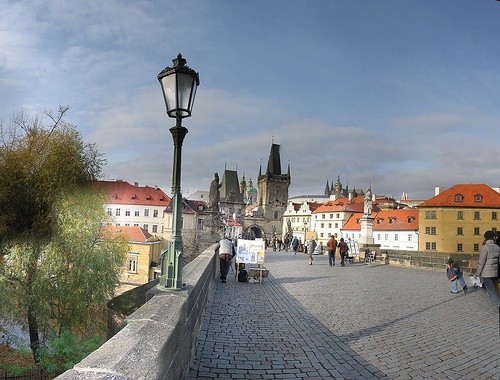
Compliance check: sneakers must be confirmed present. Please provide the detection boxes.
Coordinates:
[481,283,485,288]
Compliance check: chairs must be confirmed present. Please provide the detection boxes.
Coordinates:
[365,251,376,264]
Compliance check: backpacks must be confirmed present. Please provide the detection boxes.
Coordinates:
[238,270,248,282]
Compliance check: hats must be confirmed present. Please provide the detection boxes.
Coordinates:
[484,231,495,239]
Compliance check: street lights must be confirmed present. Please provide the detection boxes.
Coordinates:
[159,53,201,292]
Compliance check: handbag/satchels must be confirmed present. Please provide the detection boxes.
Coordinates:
[446,266,458,282]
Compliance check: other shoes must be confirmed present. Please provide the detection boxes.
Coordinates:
[450,290,458,294]
[462,286,468,294]
[329,265,335,267]
[308,262,313,265]
[340,263,345,266]
[220,276,227,283]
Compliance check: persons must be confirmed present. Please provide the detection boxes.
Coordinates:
[306,238,317,265]
[263,236,298,254]
[338,238,349,267]
[474,231,500,304]
[446,257,468,294]
[214,238,236,283]
[326,235,338,267]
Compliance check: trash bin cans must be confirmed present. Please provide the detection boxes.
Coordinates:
[359,248,369,259]
